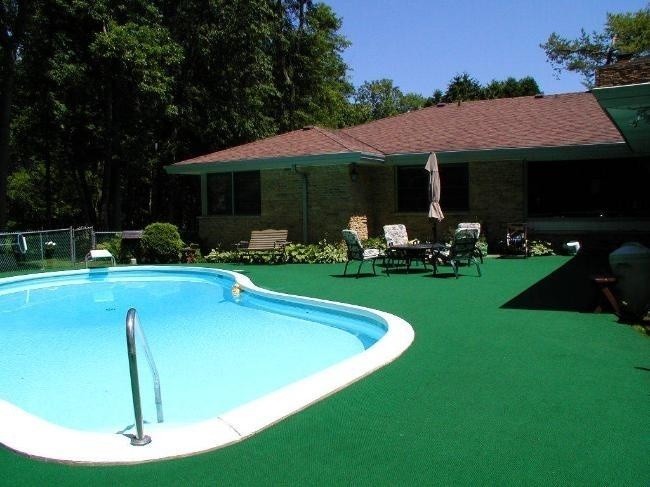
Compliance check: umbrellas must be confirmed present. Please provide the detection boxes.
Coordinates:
[423,149,446,244]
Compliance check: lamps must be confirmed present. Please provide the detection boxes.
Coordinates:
[349,162,360,181]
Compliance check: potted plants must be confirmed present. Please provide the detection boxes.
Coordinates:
[44,239,58,260]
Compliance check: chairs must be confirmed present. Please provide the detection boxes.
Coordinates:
[496,222,529,260]
[342,222,483,280]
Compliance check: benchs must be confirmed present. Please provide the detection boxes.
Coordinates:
[236,228,293,264]
